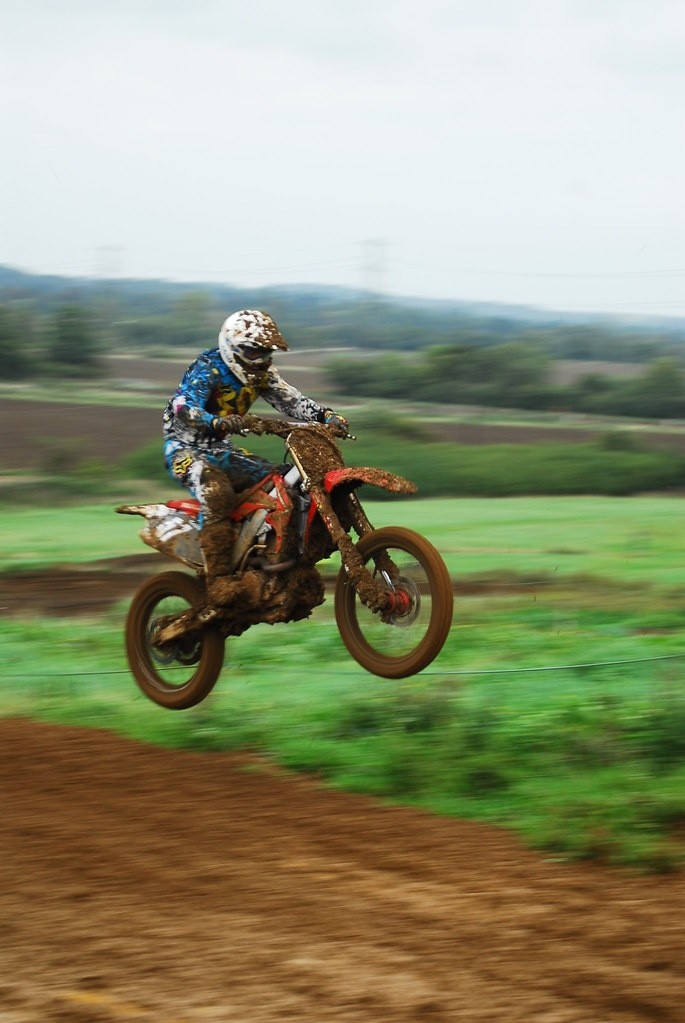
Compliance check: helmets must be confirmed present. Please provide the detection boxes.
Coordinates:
[218,309,289,389]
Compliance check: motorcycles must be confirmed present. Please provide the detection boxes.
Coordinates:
[115,413,454,710]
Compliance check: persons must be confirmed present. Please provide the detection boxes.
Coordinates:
[164,309,347,608]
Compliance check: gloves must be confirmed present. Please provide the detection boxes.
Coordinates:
[214,414,249,438]
[324,411,349,440]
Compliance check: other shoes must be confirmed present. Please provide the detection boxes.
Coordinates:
[205,575,247,608]
